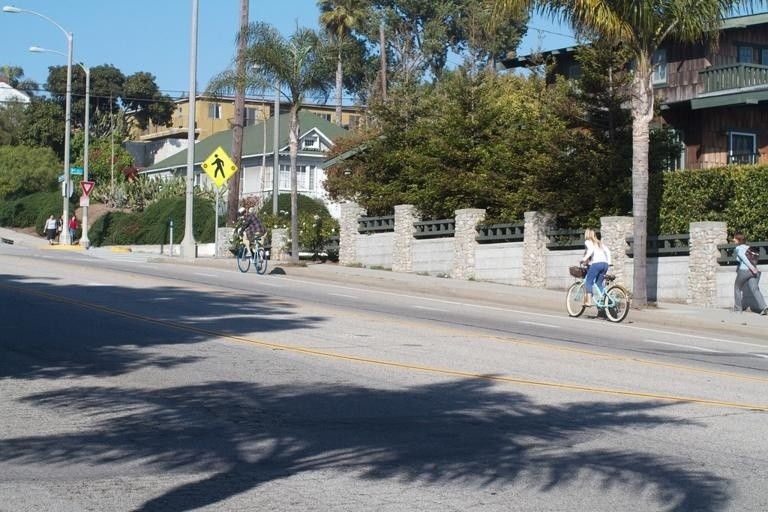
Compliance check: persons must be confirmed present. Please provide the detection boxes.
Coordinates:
[238,207,267,256]
[68,216,77,245]
[211,154,226,178]
[733,233,768,315]
[57,215,64,234]
[43,214,58,244]
[579,226,611,306]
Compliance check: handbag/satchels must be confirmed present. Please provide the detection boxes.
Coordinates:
[745,247,760,265]
[58,226,63,231]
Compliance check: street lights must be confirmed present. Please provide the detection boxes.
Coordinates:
[28,45,92,247]
[2,6,72,246]
[251,64,281,218]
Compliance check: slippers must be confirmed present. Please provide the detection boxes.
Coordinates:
[582,304,592,307]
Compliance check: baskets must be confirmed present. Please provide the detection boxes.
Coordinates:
[570,266,586,279]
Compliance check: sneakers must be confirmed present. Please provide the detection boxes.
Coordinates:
[761,306,768,314]
[246,254,252,257]
[734,307,744,312]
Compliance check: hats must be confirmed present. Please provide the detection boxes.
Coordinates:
[238,206,245,213]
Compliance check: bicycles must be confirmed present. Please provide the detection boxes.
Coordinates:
[236,234,268,275]
[566,261,630,323]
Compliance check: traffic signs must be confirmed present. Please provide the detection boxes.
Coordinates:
[57,174,65,183]
[70,168,83,176]
